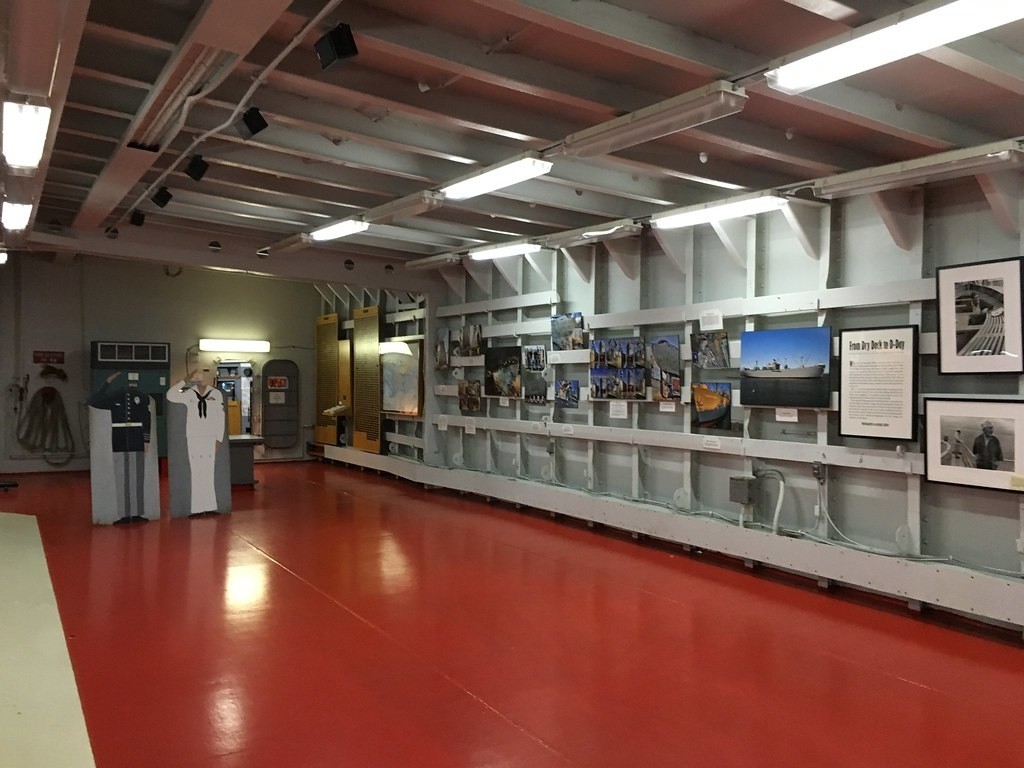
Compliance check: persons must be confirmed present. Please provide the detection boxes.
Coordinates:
[534,349,540,366]
[973,421,1003,470]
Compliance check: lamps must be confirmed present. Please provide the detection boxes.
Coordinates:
[313,22,358,70]
[812,140,1024,199]
[563,80,749,159]
[762,0,1024,95]
[468,238,542,260]
[151,186,173,209]
[207,241,221,251]
[439,151,556,200]
[1,200,33,230]
[270,233,314,255]
[650,189,790,232]
[361,190,447,225]
[105,226,118,239]
[180,154,209,182]
[0,250,8,265]
[235,107,268,140]
[256,248,269,258]
[130,211,147,226]
[405,254,463,271]
[543,220,642,250]
[311,215,371,241]
[0,91,52,176]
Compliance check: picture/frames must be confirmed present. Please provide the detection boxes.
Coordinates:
[922,396,1024,495]
[936,256,1024,375]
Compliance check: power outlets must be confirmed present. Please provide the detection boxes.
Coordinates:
[812,460,825,478]
